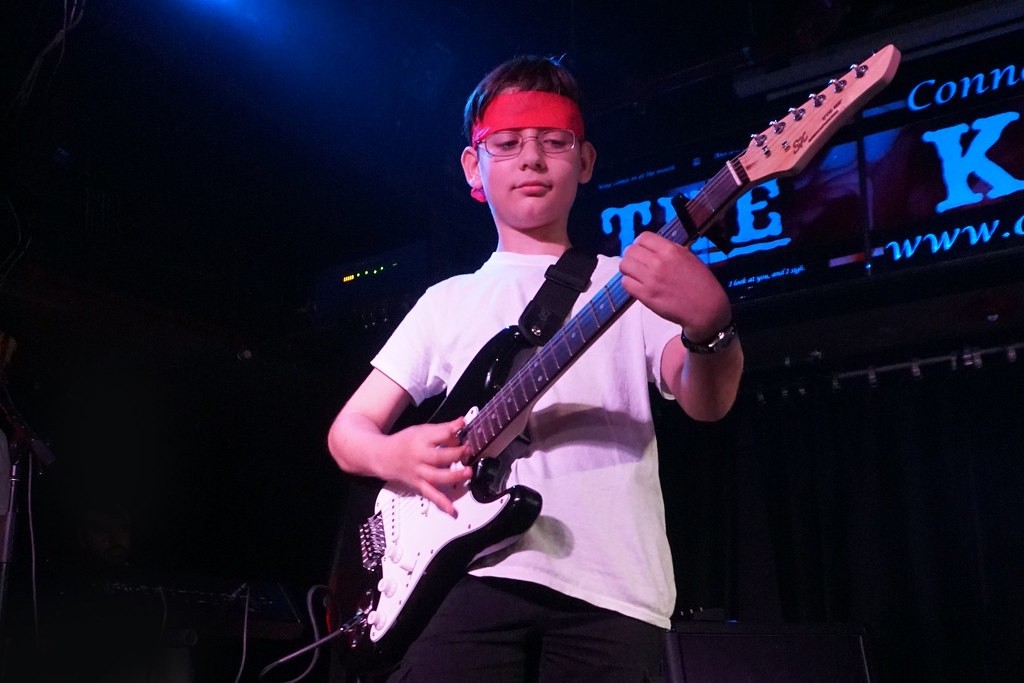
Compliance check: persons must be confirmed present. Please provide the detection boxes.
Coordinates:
[328,56,744,683]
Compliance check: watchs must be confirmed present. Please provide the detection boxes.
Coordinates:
[681,313,738,354]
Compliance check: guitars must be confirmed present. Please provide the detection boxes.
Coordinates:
[325,42,901,656]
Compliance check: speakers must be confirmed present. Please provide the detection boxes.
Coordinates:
[654,620,881,683]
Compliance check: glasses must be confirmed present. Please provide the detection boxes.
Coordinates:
[475,128,581,158]
